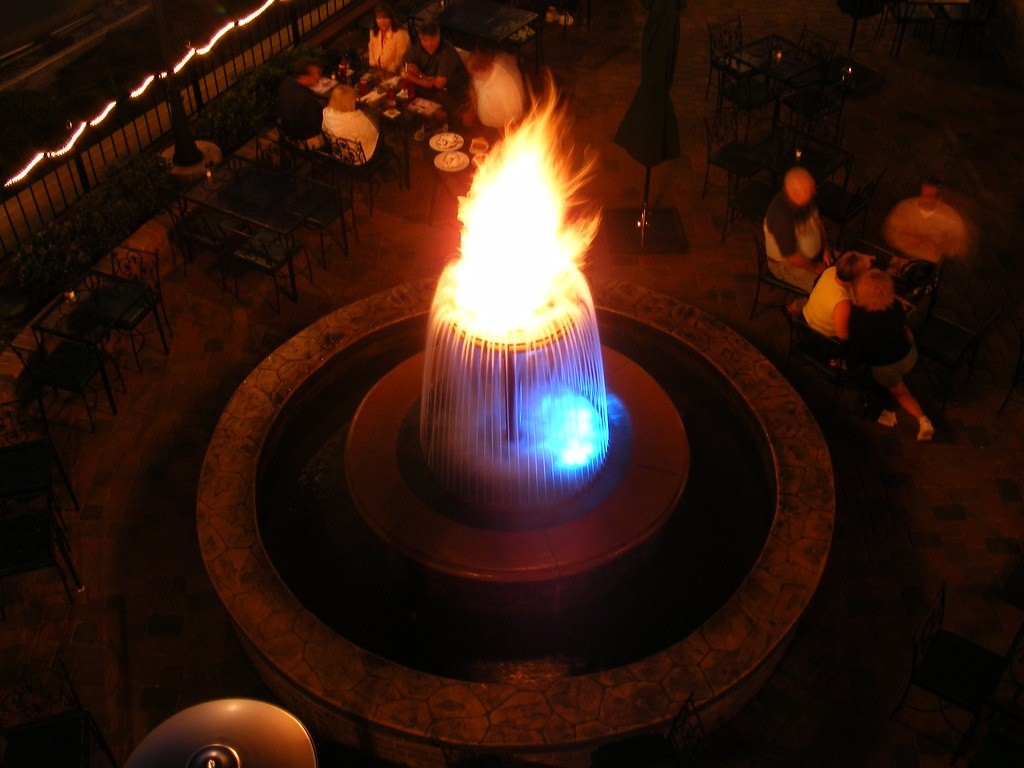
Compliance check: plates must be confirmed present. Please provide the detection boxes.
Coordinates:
[434,151,470,172]
[469,137,489,155]
[428,132,464,152]
[471,154,487,165]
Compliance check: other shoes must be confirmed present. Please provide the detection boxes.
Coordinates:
[917,421,934,441]
[413,124,428,142]
[878,410,898,428]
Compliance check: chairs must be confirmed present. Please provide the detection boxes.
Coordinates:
[505,0,596,67]
[406,15,499,139]
[0,338,125,606]
[703,17,1024,441]
[845,0,997,64]
[153,196,315,316]
[889,579,1024,768]
[0,644,120,768]
[668,692,754,768]
[226,118,403,271]
[87,242,174,373]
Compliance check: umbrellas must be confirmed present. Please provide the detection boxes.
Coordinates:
[612,0,687,253]
[837,0,888,56]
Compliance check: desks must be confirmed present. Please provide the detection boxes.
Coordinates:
[739,124,854,200]
[826,241,942,323]
[0,438,84,592]
[31,271,170,417]
[409,4,542,91]
[310,57,454,190]
[178,155,350,303]
[719,34,828,127]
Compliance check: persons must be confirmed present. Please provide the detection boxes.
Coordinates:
[278,0,470,180]
[762,166,975,441]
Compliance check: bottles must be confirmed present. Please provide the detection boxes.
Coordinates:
[387,85,396,115]
[339,58,348,86]
